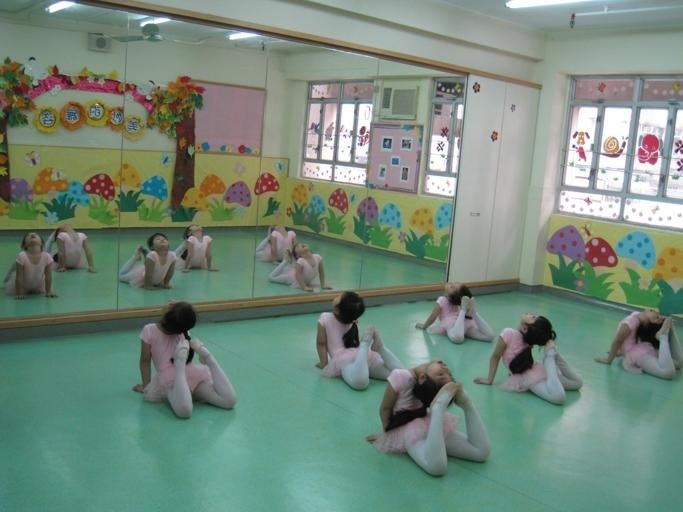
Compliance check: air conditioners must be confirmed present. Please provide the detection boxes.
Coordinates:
[88,34,112,53]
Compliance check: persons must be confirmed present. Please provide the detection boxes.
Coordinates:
[473,312,582,405]
[415,281,494,344]
[132,299,236,418]
[315,291,404,390]
[119,232,178,290]
[269,242,334,291]
[45,223,96,272]
[366,359,490,476]
[174,224,220,272]
[256,223,296,265]
[593,308,683,380]
[4,231,58,299]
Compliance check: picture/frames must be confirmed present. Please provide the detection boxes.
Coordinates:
[380,136,394,152]
[400,166,411,183]
[390,156,400,166]
[400,137,413,152]
[377,164,387,180]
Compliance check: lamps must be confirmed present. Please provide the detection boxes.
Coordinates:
[139,16,171,28]
[44,1,76,14]
[224,32,261,40]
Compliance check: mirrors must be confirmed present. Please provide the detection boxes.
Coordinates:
[0,0,469,330]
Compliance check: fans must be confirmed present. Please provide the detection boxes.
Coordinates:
[110,23,201,46]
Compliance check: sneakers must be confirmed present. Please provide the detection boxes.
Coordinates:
[173,339,190,368]
[543,339,556,364]
[461,296,470,314]
[466,297,474,317]
[668,317,675,342]
[371,329,383,351]
[359,326,375,351]
[268,227,272,240]
[655,318,670,340]
[282,248,292,264]
[133,246,142,261]
[190,337,212,364]
[455,384,466,408]
[142,250,150,265]
[430,382,457,411]
